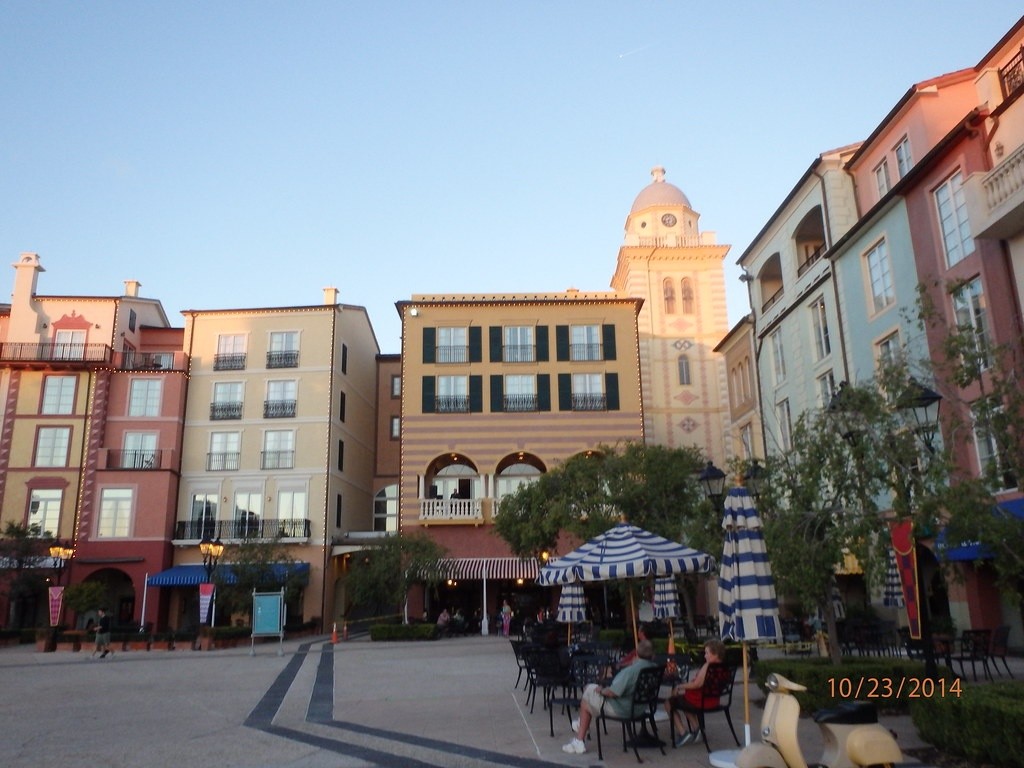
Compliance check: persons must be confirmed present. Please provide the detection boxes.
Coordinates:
[562,622,657,754]
[538,607,554,625]
[437,606,490,633]
[91,609,114,659]
[749,640,759,665]
[664,640,724,747]
[501,599,511,635]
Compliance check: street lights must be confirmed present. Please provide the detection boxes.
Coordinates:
[43,534,77,652]
[198,534,226,583]
[832,376,946,680]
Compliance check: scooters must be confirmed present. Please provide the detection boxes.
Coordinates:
[735,671,903,768]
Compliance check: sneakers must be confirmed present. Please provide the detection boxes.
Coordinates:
[675,731,691,747]
[562,737,586,754]
[572,717,590,734]
[693,727,702,743]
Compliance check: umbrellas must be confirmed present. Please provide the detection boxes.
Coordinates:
[555,584,588,643]
[809,572,845,635]
[884,531,905,639]
[652,575,681,648]
[534,522,719,650]
[719,476,783,747]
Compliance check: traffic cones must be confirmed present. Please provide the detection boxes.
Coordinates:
[328,621,341,644]
[341,620,349,641]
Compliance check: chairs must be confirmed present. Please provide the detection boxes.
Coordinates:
[778,614,1016,683]
[505,612,743,764]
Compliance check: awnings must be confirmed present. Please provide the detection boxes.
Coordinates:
[405,557,564,580]
[832,532,875,575]
[930,496,1024,565]
[146,563,308,588]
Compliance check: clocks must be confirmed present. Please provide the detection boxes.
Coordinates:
[661,213,678,228]
